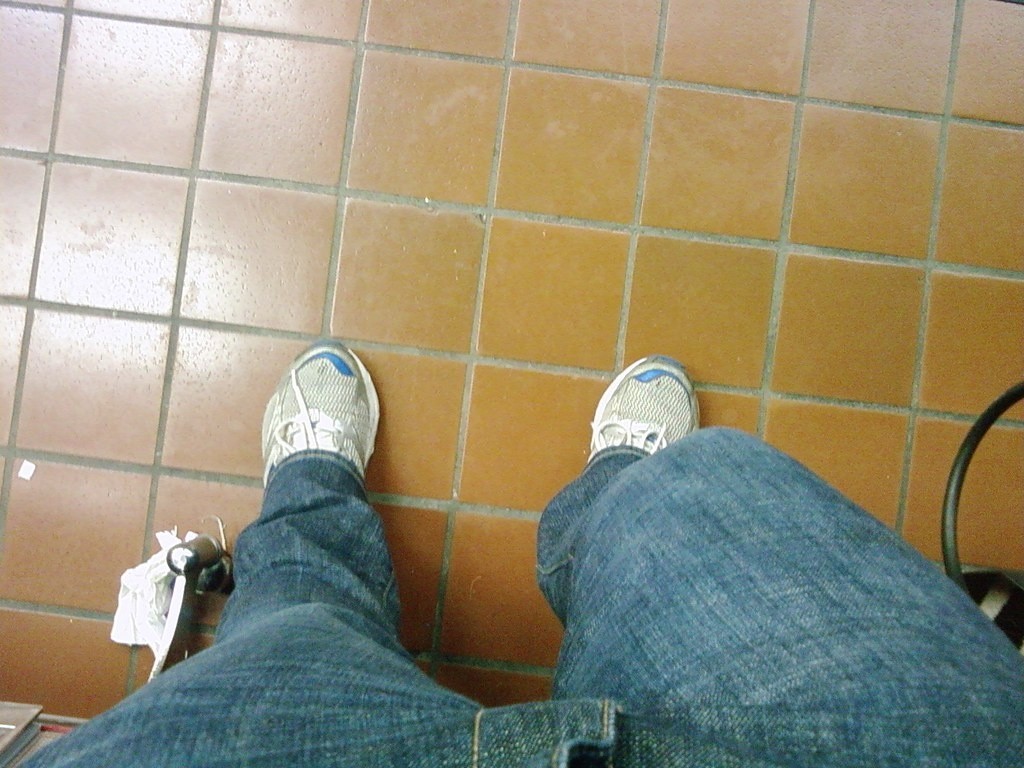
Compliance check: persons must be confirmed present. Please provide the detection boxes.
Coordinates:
[20,338,1024,768]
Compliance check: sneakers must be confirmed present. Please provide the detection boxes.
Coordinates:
[260,339,380,491]
[587,355,701,463]
